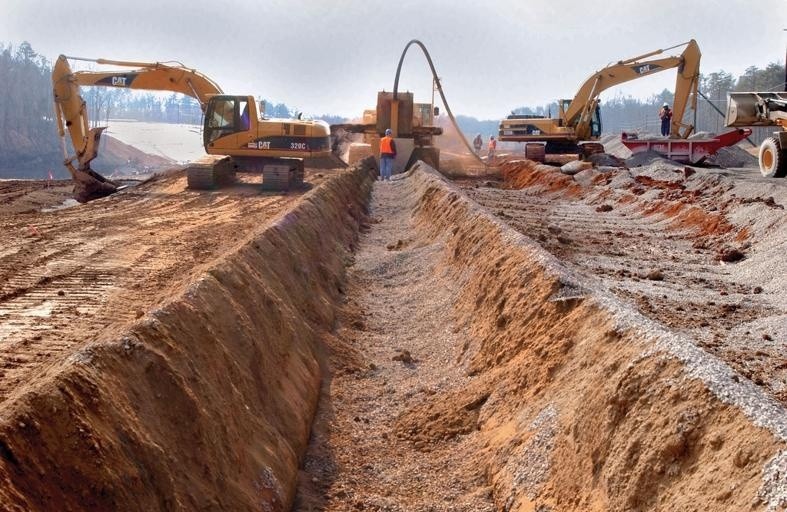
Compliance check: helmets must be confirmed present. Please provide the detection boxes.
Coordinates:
[385,129,391,134]
[662,103,668,107]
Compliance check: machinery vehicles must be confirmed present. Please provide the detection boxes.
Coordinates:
[348,75,441,173]
[497,40,701,167]
[722,92,786,179]
[51,54,332,204]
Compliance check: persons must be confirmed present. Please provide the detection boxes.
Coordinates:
[379,129,397,180]
[658,103,672,137]
[486,135,496,160]
[472,133,482,157]
